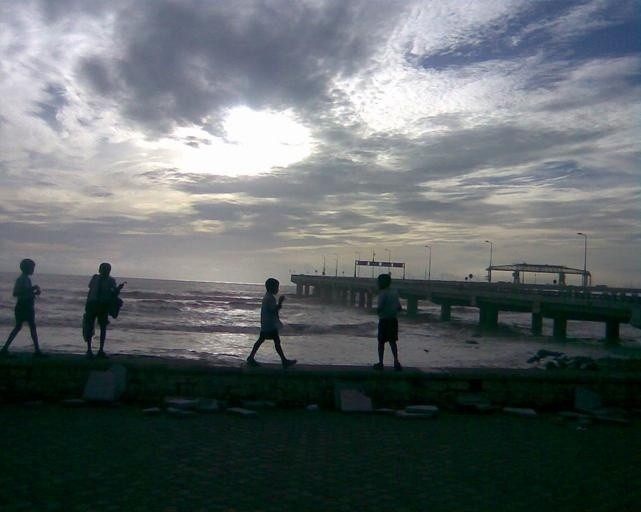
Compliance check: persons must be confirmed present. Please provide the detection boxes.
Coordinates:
[247,278,298,366]
[371,273,403,368]
[1,258,48,357]
[83,262,125,357]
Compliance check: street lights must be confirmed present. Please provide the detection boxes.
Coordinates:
[108,297,124,320]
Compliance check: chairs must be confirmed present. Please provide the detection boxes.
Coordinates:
[86,349,107,356]
[372,362,402,370]
[247,356,297,367]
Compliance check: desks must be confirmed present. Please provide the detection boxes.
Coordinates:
[577,232,587,294]
[424,245,431,280]
[484,240,493,284]
[384,248,391,276]
